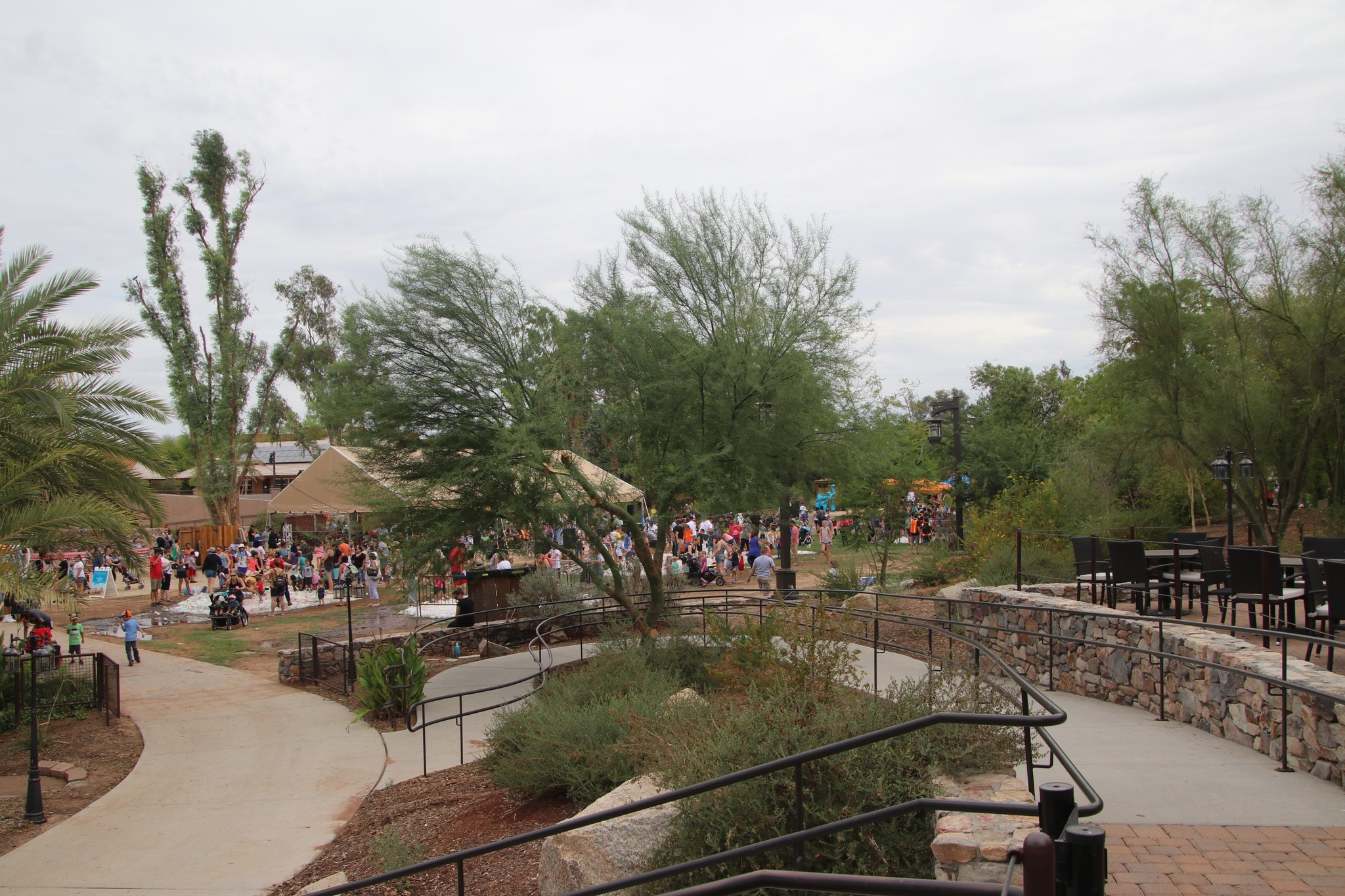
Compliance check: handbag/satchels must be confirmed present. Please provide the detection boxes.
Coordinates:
[366,561,382,580]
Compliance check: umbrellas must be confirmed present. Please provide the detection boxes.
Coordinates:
[877,472,978,505]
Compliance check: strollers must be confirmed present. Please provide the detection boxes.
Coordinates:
[116,562,145,590]
[680,552,724,588]
[208,588,250,630]
[797,523,814,545]
[80,568,92,595]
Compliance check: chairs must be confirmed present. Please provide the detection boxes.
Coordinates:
[1161,536,1227,612]
[1165,531,1208,606]
[1227,545,1305,646]
[1071,536,1113,605]
[1302,536,1345,560]
[1106,540,1175,615]
[1321,558,1345,672]
[1196,538,1232,629]
[1301,550,1329,662]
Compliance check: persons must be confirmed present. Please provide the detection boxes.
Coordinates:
[132,538,141,549]
[15,540,142,603]
[447,587,475,628]
[425,500,838,601]
[118,610,145,666]
[3,561,18,623]
[66,614,85,665]
[27,620,60,670]
[839,502,976,554]
[21,608,53,654]
[148,520,425,615]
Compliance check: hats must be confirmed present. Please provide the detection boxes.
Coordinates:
[228,594,236,599]
[823,514,830,517]
[70,613,80,620]
[473,558,487,569]
[42,621,53,629]
[649,520,653,525]
[238,544,246,549]
[732,530,739,535]
[207,547,216,552]
[118,610,132,618]
[680,521,686,524]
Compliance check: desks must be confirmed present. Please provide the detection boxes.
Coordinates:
[1144,550,1200,616]
[1270,557,1319,637]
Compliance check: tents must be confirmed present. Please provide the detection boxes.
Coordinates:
[265,445,645,550]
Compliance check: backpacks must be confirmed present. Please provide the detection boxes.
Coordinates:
[341,564,359,580]
[922,523,931,534]
[271,568,287,589]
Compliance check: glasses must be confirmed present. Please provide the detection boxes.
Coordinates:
[355,549,360,551]
[230,598,234,600]
[217,548,229,551]
[801,517,803,518]
[230,575,236,577]
[155,551,185,556]
[454,595,458,599]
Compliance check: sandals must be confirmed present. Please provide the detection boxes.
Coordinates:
[366,603,380,607]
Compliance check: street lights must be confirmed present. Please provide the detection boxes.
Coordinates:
[918,393,964,549]
[1211,447,1254,548]
[2,630,57,826]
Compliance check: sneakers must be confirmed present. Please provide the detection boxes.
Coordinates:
[268,613,285,616]
[69,660,75,665]
[151,592,194,606]
[137,660,140,663]
[79,660,85,665]
[129,662,133,666]
[699,575,738,588]
